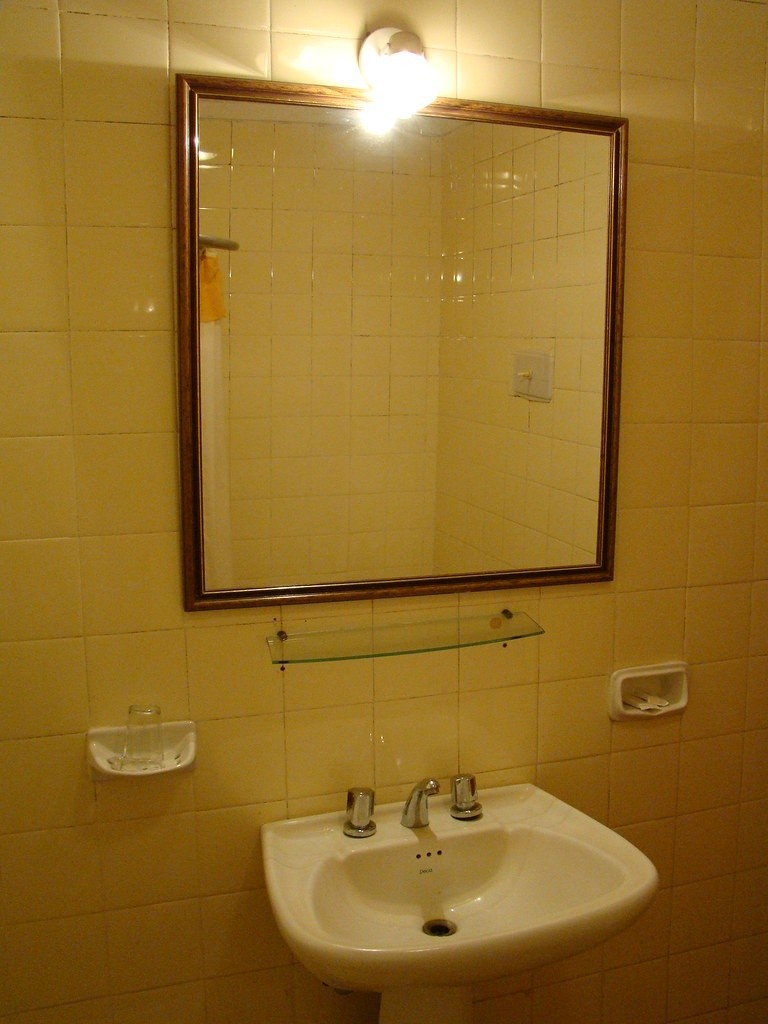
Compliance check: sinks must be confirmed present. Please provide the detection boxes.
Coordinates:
[261,803,662,989]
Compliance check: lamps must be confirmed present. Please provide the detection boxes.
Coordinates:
[357,25,436,121]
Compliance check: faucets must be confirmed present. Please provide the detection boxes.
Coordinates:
[398,776,441,830]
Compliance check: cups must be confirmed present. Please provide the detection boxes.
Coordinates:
[123,704,164,764]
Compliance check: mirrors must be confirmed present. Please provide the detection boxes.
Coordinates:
[174,73,631,612]
